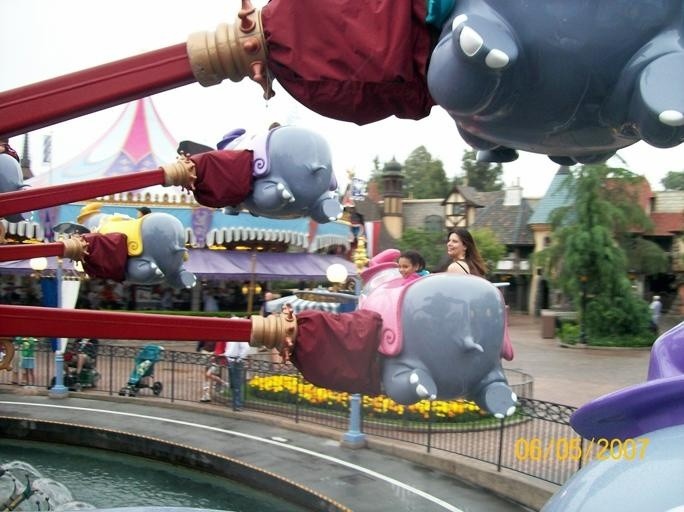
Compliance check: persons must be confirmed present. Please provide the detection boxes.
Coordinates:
[399,252,430,285]
[648,295,664,327]
[136,207,153,220]
[62,337,96,390]
[446,227,488,280]
[12,335,39,387]
[199,341,250,412]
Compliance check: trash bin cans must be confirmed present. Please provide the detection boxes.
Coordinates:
[538,308,557,340]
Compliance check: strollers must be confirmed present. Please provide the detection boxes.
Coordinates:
[119,343,166,397]
